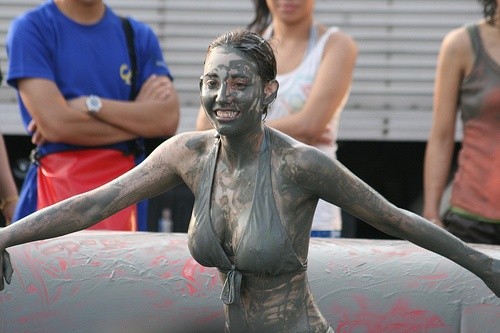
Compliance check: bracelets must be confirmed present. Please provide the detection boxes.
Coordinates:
[0,194,20,210]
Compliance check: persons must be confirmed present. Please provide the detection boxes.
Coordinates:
[422,0,500,245]
[196,0,358,238]
[0,30,500,332]
[0,65,20,227]
[5,0,180,232]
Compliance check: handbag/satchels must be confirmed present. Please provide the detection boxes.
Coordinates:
[36,150,138,232]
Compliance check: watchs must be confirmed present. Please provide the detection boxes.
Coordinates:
[85,94,103,116]
